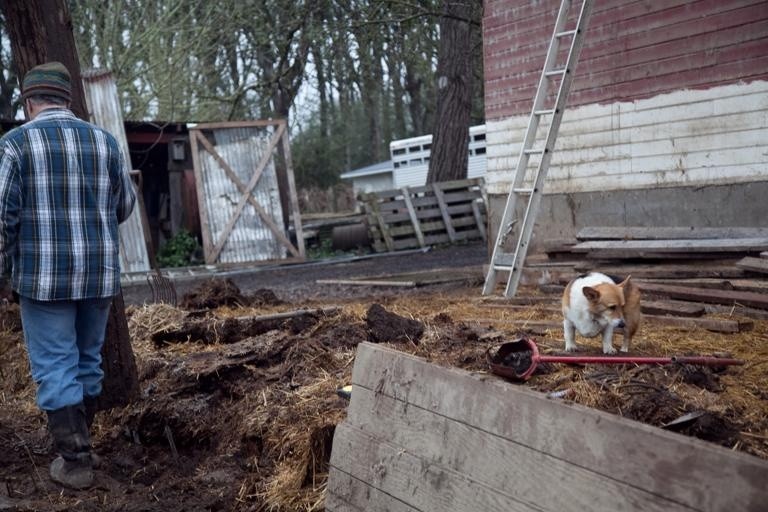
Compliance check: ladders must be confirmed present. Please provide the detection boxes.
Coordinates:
[481,0,594,298]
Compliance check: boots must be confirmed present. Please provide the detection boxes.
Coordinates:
[48,397,113,488]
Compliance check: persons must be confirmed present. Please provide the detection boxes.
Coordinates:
[0,60,137,491]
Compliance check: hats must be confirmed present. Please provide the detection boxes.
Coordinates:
[18,61,73,102]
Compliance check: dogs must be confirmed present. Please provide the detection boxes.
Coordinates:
[561,271,642,355]
